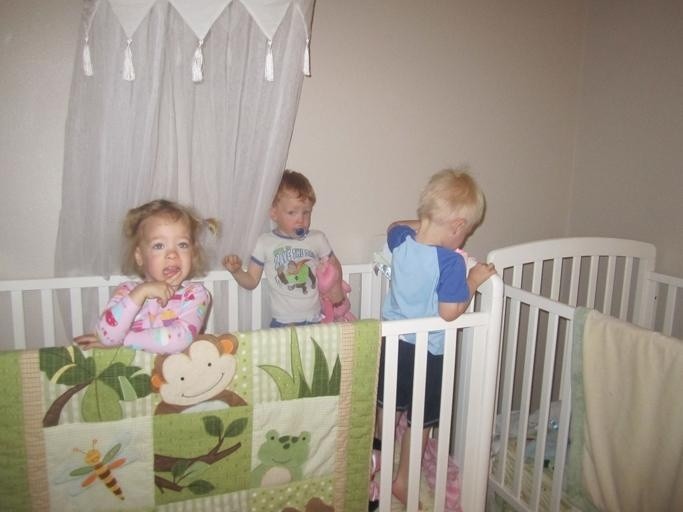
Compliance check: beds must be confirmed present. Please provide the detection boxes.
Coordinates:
[484,237,683,512]
[0,236,504,512]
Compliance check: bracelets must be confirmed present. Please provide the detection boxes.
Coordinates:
[330,297,346,309]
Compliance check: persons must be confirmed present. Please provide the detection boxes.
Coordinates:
[73,198,221,354]
[372,169,499,512]
[222,170,345,329]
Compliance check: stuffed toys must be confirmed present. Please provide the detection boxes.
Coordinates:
[316,257,357,323]
